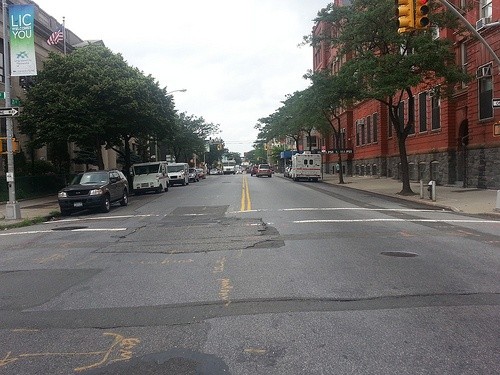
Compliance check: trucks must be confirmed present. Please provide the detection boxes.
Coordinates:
[290,151,321,181]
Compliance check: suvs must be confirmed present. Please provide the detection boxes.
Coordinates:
[129,161,169,194]
[166,162,189,186]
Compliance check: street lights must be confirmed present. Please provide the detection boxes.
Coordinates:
[154,88,188,162]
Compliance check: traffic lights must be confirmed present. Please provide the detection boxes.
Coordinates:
[414,0,429,30]
[395,0,414,34]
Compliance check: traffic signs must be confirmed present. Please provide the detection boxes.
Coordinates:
[0,107,20,118]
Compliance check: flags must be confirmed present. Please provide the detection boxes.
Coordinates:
[47,24,63,45]
[8,4,37,77]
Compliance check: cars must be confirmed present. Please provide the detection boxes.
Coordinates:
[197,169,205,178]
[222,160,272,177]
[284,167,292,177]
[189,168,198,182]
[57,170,130,215]
